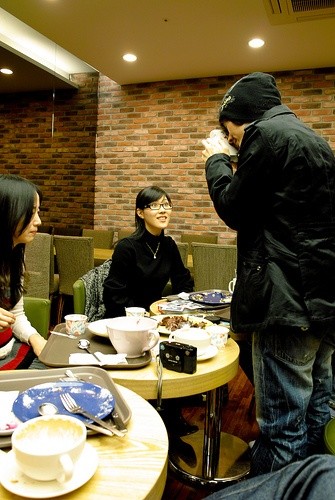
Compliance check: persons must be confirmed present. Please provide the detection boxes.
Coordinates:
[203,454,335,500]
[103,186,198,436]
[202,71,335,478]
[0,174,47,370]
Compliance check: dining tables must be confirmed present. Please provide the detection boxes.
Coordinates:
[0,383,170,500]
[104,338,240,399]
[149,289,249,487]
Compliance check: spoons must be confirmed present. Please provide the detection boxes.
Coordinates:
[78,339,102,363]
[39,402,114,437]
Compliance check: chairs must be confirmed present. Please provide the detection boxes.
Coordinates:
[23,226,237,339]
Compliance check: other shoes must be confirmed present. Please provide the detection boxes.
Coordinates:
[175,414,199,437]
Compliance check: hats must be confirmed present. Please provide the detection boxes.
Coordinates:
[218,72,282,120]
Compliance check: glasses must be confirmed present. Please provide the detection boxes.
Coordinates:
[144,203,173,210]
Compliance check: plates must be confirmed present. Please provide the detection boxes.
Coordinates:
[150,315,214,334]
[197,345,219,361]
[0,442,99,499]
[12,381,115,424]
[88,316,126,337]
[0,391,24,435]
[189,292,231,305]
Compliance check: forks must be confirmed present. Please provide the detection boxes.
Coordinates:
[60,393,125,438]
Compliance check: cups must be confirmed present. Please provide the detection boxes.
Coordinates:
[169,328,212,356]
[11,414,87,484]
[106,316,160,358]
[228,278,237,294]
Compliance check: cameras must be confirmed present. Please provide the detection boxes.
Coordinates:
[160,340,196,374]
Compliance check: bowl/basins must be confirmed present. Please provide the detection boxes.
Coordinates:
[205,326,230,346]
[64,314,87,335]
[125,307,145,317]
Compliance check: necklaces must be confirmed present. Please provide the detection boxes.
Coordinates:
[146,242,160,259]
